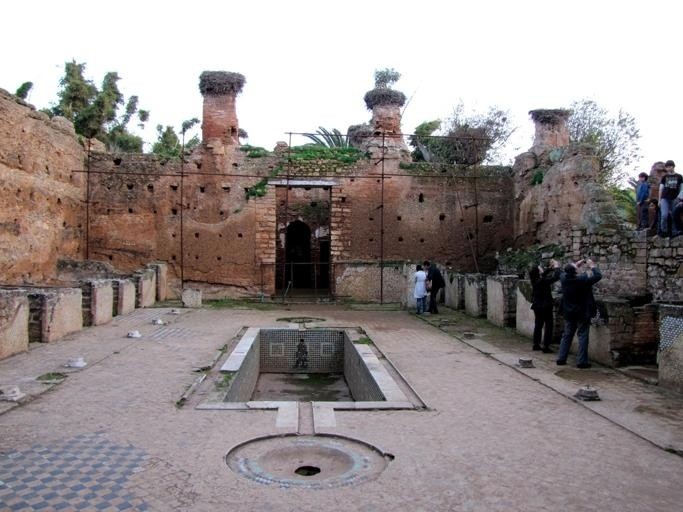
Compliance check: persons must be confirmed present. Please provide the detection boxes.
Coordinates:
[636,159,683,239]
[528,259,561,354]
[556,258,602,369]
[412,261,446,314]
